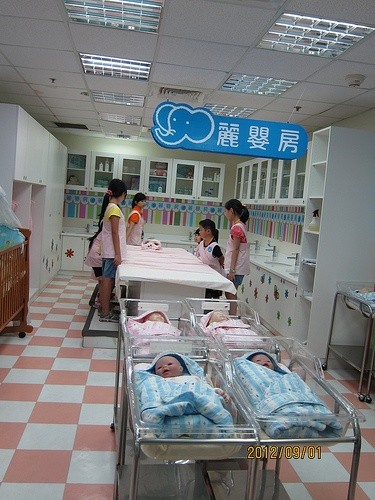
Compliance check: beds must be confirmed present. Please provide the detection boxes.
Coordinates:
[0,227,34,335]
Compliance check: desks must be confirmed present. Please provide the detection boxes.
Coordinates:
[111,244,235,325]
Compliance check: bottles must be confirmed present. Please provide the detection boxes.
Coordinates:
[99,159,114,171]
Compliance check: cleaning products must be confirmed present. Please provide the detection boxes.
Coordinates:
[309,209,320,232]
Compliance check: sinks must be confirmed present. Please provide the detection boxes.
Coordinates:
[289,272,300,277]
[264,261,293,270]
[251,254,272,261]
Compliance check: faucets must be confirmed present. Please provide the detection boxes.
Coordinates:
[265,244,277,257]
[287,252,300,266]
[249,240,260,253]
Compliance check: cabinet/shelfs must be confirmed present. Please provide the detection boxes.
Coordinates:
[33,123,50,186]
[15,106,35,182]
[268,157,295,204]
[291,126,374,358]
[170,159,225,202]
[313,290,375,403]
[250,158,271,204]
[291,142,312,204]
[60,237,97,271]
[62,152,118,192]
[234,162,252,204]
[117,155,172,198]
[31,134,69,286]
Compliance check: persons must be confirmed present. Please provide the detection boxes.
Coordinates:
[235,350,344,437]
[130,310,183,354]
[86,218,104,310]
[224,199,250,316]
[126,192,146,245]
[97,179,126,321]
[130,352,234,440]
[193,219,224,314]
[205,310,264,347]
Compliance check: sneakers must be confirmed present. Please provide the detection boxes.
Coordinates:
[98,307,119,323]
